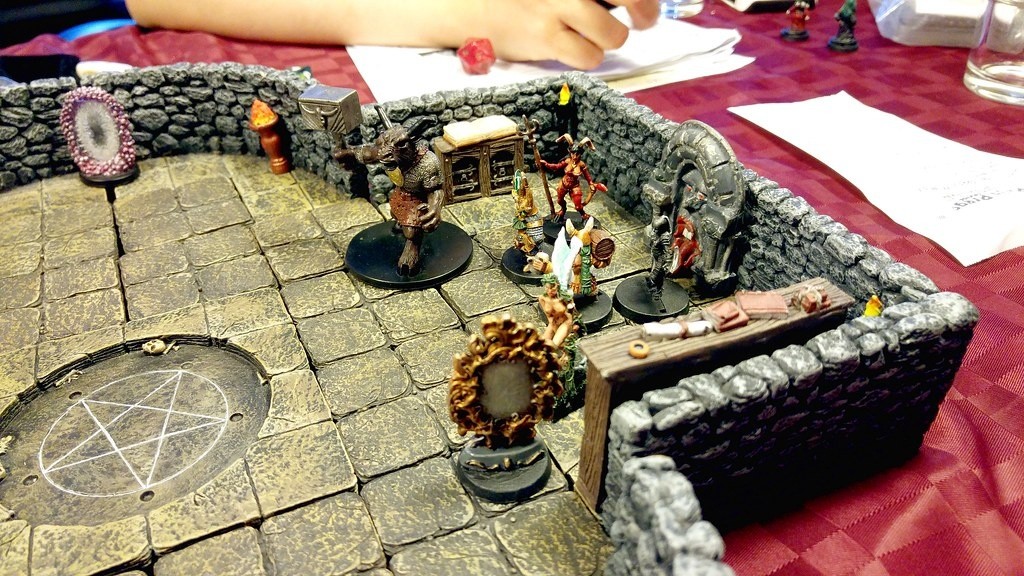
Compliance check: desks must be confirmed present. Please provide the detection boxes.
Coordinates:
[1,0,1024,576]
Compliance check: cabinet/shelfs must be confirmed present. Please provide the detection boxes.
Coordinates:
[580,277,856,511]
[433,135,524,204]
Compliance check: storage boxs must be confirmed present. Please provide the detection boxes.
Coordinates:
[299,86,364,135]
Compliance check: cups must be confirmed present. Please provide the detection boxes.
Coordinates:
[963,0,1024,107]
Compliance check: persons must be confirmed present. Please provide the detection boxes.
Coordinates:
[511,106,672,348]
[123,0,660,71]
